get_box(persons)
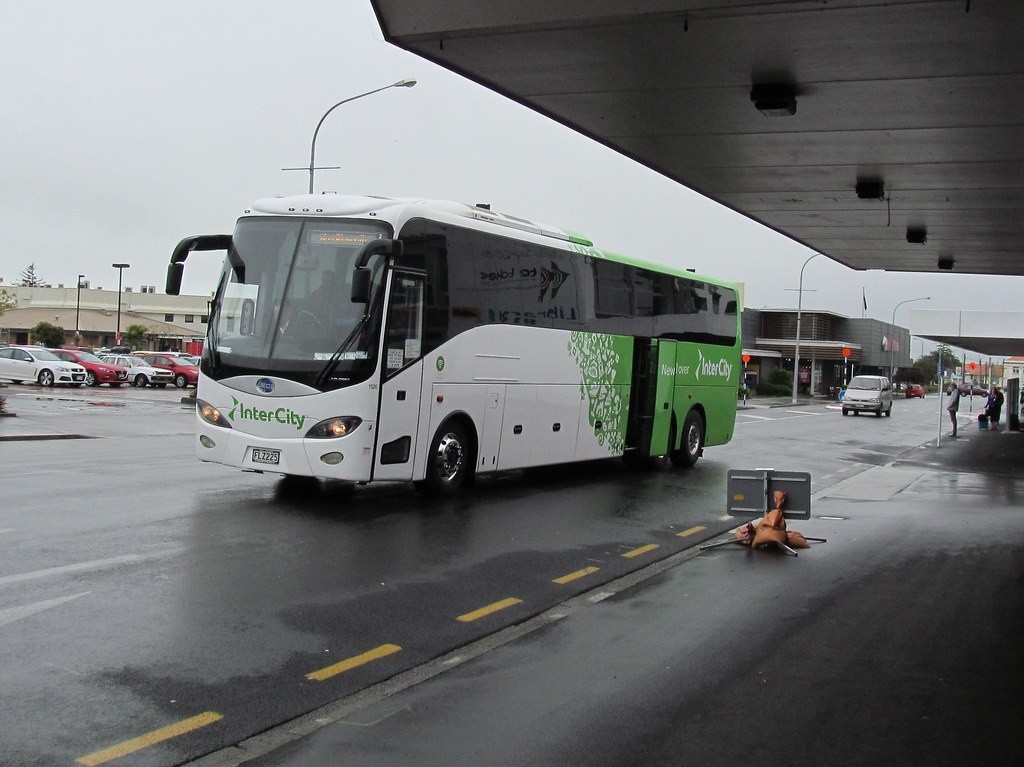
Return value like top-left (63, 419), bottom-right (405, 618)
top-left (947, 382), bottom-right (960, 436)
top-left (984, 387), bottom-right (1001, 431)
top-left (996, 386), bottom-right (1004, 424)
top-left (262, 298), bottom-right (306, 344)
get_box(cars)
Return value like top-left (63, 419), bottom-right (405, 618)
top-left (982, 384), bottom-right (1001, 390)
top-left (97, 356), bottom-right (175, 388)
top-left (959, 384), bottom-right (988, 397)
top-left (59, 347), bottom-right (201, 365)
top-left (47, 348), bottom-right (130, 388)
top-left (0, 346), bottom-right (87, 387)
top-left (140, 353), bottom-right (200, 388)
top-left (947, 386), bottom-right (953, 395)
top-left (906, 384), bottom-right (925, 398)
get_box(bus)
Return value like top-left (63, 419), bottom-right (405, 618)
top-left (165, 193), bottom-right (743, 504)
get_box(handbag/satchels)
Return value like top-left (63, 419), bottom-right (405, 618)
top-left (986, 410), bottom-right (990, 417)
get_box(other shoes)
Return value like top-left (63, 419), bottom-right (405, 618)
top-left (949, 434), bottom-right (956, 436)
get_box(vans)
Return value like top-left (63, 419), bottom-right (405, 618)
top-left (841, 375), bottom-right (893, 415)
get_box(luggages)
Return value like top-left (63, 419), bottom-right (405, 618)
top-left (979, 408), bottom-right (989, 431)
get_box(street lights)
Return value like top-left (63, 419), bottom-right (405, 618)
top-left (76, 275), bottom-right (87, 347)
top-left (890, 297), bottom-right (931, 384)
top-left (309, 77), bottom-right (418, 192)
top-left (113, 263), bottom-right (130, 346)
top-left (913, 337), bottom-right (924, 357)
